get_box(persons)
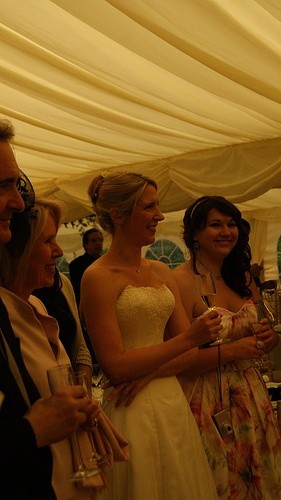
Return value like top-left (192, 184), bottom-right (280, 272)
top-left (0, 118), bottom-right (281, 500)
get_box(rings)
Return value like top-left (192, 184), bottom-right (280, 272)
top-left (255, 341), bottom-right (264, 349)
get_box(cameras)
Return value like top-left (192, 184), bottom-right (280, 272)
top-left (212, 409), bottom-right (234, 437)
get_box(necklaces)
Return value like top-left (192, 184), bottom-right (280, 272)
top-left (109, 248), bottom-right (143, 273)
top-left (196, 257), bottom-right (222, 280)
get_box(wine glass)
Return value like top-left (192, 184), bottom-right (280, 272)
top-left (245, 304), bottom-right (267, 367)
top-left (195, 271), bottom-right (231, 346)
top-left (69, 369), bottom-right (112, 464)
top-left (46, 362), bottom-right (100, 482)
